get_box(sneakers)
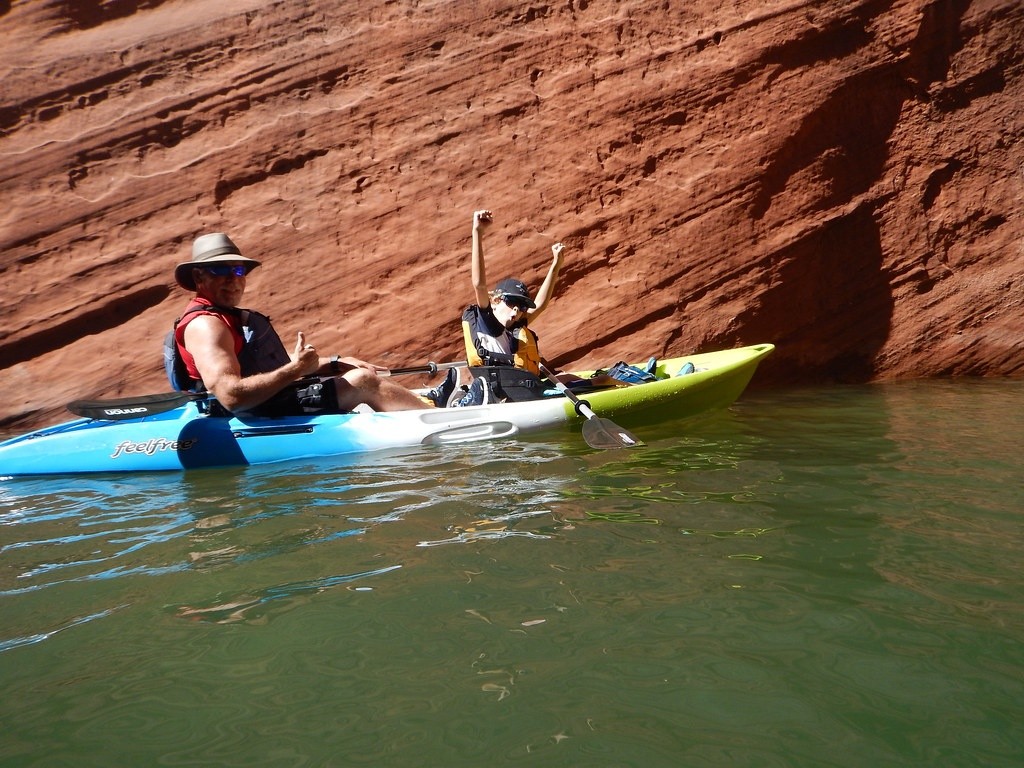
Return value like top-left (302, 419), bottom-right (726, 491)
top-left (421, 366), bottom-right (461, 408)
top-left (451, 376), bottom-right (488, 407)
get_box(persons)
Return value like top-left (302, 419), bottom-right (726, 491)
top-left (164, 232), bottom-right (489, 420)
top-left (460, 209), bottom-right (694, 407)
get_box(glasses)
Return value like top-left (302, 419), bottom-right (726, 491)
top-left (499, 295), bottom-right (527, 313)
top-left (198, 263), bottom-right (248, 276)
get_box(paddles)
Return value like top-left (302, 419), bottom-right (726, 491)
top-left (67, 360), bottom-right (470, 422)
top-left (539, 361), bottom-right (646, 449)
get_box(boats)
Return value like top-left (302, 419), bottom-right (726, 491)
top-left (0, 339), bottom-right (776, 481)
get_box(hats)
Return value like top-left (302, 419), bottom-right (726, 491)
top-left (494, 279), bottom-right (536, 309)
top-left (174, 233), bottom-right (263, 292)
